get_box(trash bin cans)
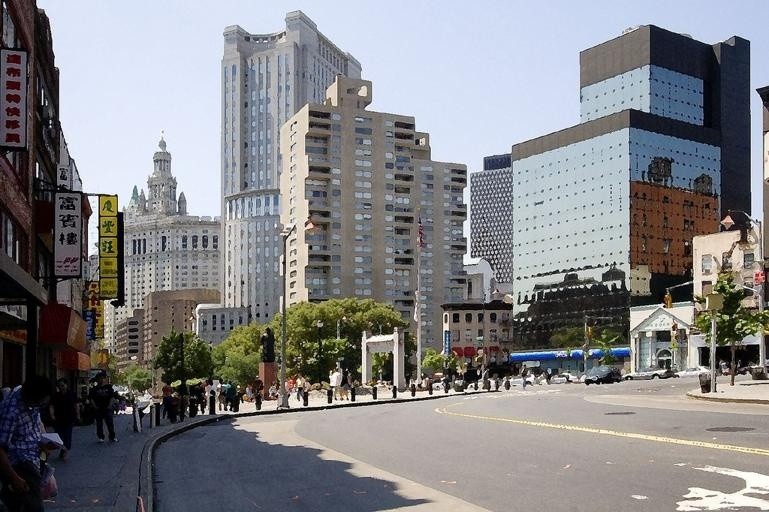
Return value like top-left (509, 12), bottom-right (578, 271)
top-left (698, 372), bottom-right (717, 393)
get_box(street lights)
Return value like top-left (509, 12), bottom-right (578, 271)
top-left (277, 217), bottom-right (315, 408)
top-left (482, 286), bottom-right (501, 371)
top-left (336, 315), bottom-right (391, 381)
top-left (317, 320), bottom-right (324, 383)
top-left (721, 209), bottom-right (767, 377)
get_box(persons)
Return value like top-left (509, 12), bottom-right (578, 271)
top-left (161, 368), bottom-right (350, 418)
top-left (89, 373), bottom-right (119, 442)
top-left (2, 377), bottom-right (81, 510)
top-left (409, 364), bottom-right (530, 391)
top-left (260, 327), bottom-right (275, 362)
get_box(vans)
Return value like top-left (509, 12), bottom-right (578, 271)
top-left (526, 366), bottom-right (551, 382)
top-left (721, 359), bottom-right (747, 376)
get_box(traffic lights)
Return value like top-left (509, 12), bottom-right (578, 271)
top-left (663, 294), bottom-right (672, 308)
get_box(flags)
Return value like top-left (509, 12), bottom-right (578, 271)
top-left (418, 216), bottom-right (426, 248)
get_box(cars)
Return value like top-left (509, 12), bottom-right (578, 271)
top-left (673, 365), bottom-right (718, 377)
top-left (584, 366), bottom-right (622, 384)
top-left (622, 367), bottom-right (675, 380)
top-left (562, 370), bottom-right (573, 375)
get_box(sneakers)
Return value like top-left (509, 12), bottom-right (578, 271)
top-left (97, 438), bottom-right (120, 442)
